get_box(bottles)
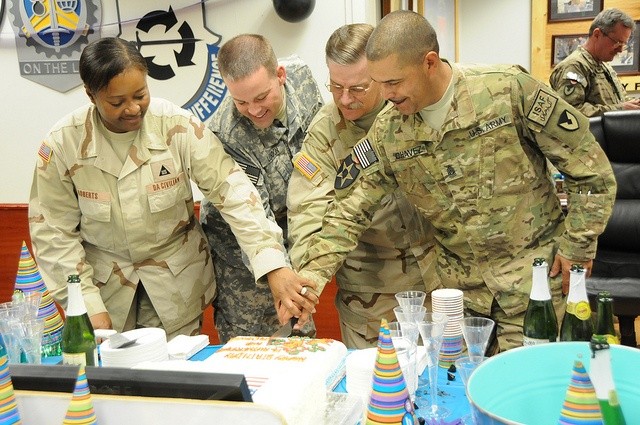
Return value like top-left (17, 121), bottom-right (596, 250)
top-left (522, 258), bottom-right (561, 347)
top-left (61, 274), bottom-right (98, 367)
top-left (591, 292), bottom-right (621, 345)
top-left (559, 264), bottom-right (595, 341)
top-left (588, 338), bottom-right (628, 425)
top-left (598, 291), bottom-right (610, 304)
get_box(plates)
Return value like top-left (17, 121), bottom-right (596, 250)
top-left (100, 326), bottom-right (168, 370)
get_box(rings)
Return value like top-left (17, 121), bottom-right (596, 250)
top-left (299, 286), bottom-right (307, 295)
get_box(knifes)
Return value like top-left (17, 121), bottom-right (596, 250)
top-left (267, 314), bottom-right (301, 346)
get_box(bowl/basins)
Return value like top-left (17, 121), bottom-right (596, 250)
top-left (463, 340), bottom-right (640, 425)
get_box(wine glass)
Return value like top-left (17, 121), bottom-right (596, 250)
top-left (0, 309), bottom-right (27, 366)
top-left (453, 356), bottom-right (487, 425)
top-left (391, 337), bottom-right (429, 412)
top-left (394, 290), bottom-right (426, 313)
top-left (393, 304), bottom-right (427, 344)
top-left (387, 321), bottom-right (430, 388)
top-left (0, 301), bottom-right (25, 312)
top-left (414, 312), bottom-right (451, 419)
top-left (11, 292), bottom-right (43, 321)
top-left (459, 316), bottom-right (495, 363)
top-left (11, 317), bottom-right (45, 365)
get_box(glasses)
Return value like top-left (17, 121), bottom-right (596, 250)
top-left (324, 71), bottom-right (373, 96)
top-left (596, 27), bottom-right (629, 50)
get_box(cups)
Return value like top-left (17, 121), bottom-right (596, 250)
top-left (431, 288), bottom-right (464, 370)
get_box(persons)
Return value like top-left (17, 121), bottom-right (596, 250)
top-left (549, 9), bottom-right (640, 118)
top-left (276, 8), bottom-right (618, 354)
top-left (198, 33), bottom-right (326, 345)
top-left (26, 37), bottom-right (321, 347)
top-left (285, 22), bottom-right (433, 350)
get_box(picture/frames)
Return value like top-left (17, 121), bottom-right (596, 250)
top-left (547, 0), bottom-right (604, 24)
top-left (551, 34), bottom-right (590, 69)
top-left (606, 20), bottom-right (640, 76)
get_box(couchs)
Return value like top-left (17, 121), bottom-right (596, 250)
top-left (588, 112), bottom-right (640, 349)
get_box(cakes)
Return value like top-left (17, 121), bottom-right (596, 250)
top-left (203, 336), bottom-right (348, 396)
